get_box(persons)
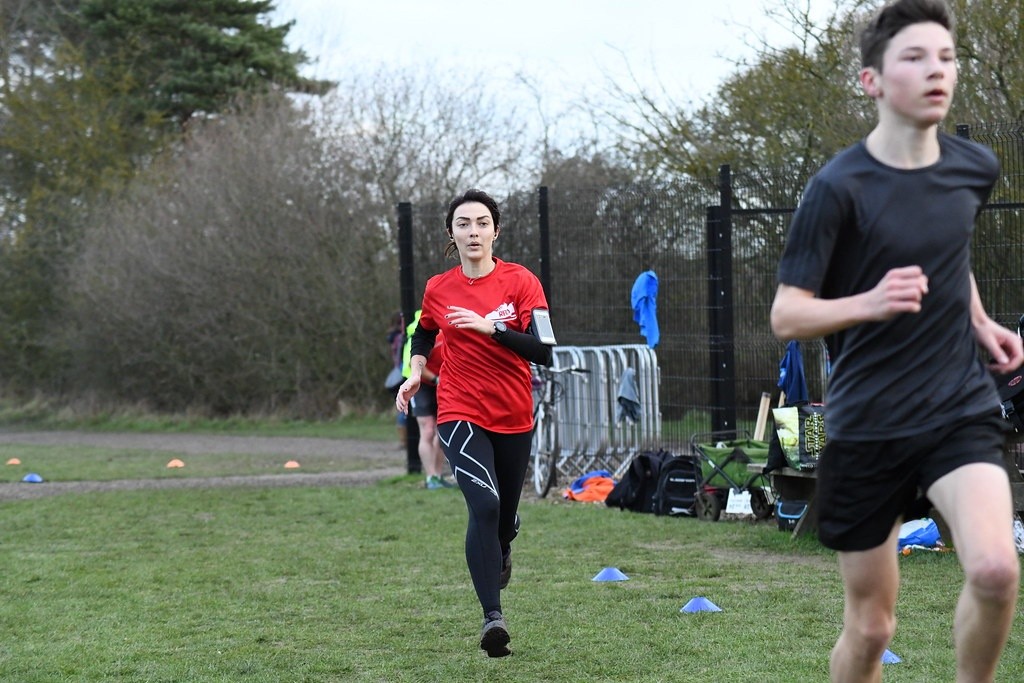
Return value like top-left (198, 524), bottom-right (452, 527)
top-left (388, 189), bottom-right (557, 658)
top-left (770, 1), bottom-right (1023, 682)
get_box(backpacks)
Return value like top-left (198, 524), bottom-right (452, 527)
top-left (605, 446), bottom-right (674, 512)
top-left (652, 456), bottom-right (705, 517)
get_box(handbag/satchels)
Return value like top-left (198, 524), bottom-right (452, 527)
top-left (773, 499), bottom-right (816, 533)
top-left (772, 402), bottom-right (827, 472)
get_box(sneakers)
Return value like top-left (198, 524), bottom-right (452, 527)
top-left (424, 475), bottom-right (443, 489)
top-left (499, 542), bottom-right (513, 590)
top-left (479, 611), bottom-right (512, 657)
top-left (438, 472), bottom-right (462, 490)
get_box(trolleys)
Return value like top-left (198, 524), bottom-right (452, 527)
top-left (690, 428), bottom-right (771, 522)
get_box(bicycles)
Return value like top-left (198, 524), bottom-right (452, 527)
top-left (529, 361), bottom-right (593, 498)
